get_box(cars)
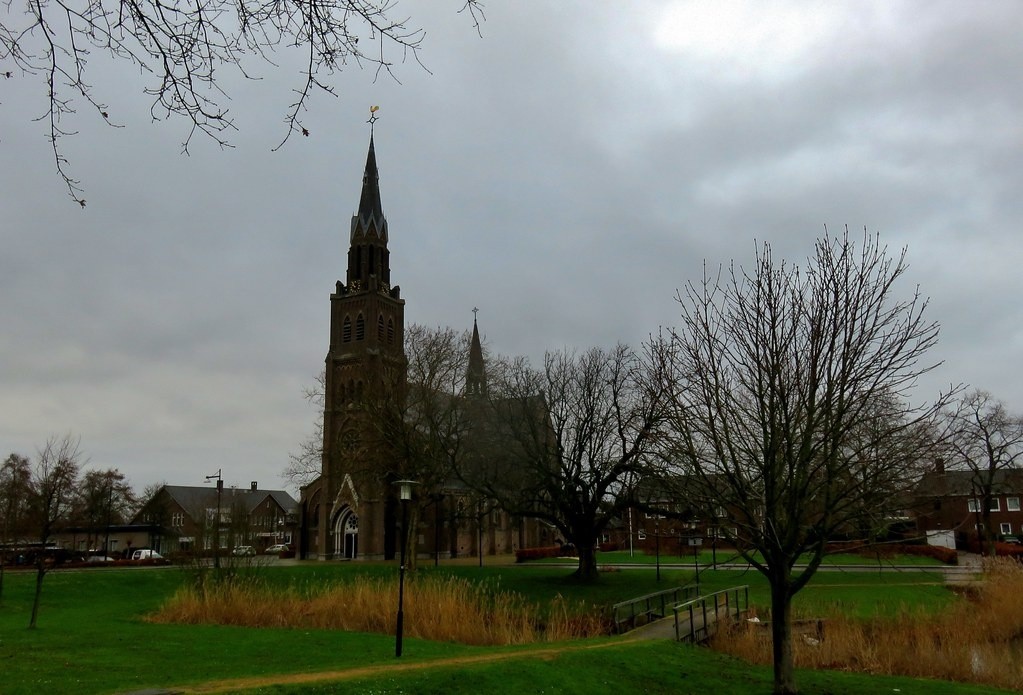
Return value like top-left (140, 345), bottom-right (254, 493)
top-left (1003, 536), bottom-right (1021, 545)
top-left (88, 556), bottom-right (114, 561)
top-left (132, 550), bottom-right (164, 560)
top-left (265, 544), bottom-right (289, 552)
top-left (233, 546), bottom-right (256, 556)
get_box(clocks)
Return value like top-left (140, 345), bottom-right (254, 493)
top-left (351, 280), bottom-right (360, 292)
top-left (380, 281), bottom-right (389, 294)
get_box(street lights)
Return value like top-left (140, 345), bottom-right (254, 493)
top-left (204, 474), bottom-right (223, 566)
top-left (389, 479), bottom-right (420, 657)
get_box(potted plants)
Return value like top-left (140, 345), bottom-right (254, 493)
top-left (279, 550), bottom-right (296, 558)
